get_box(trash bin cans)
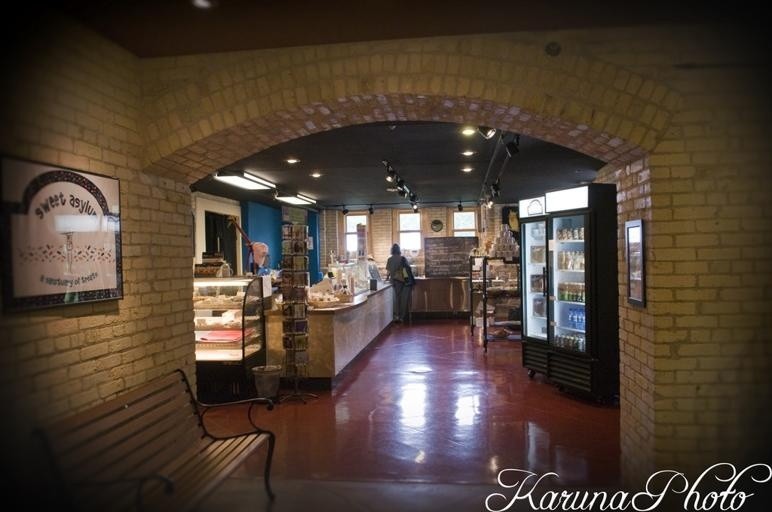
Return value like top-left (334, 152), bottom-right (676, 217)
top-left (251, 364), bottom-right (282, 404)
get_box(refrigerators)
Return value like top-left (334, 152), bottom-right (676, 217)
top-left (544, 182), bottom-right (617, 402)
top-left (517, 194), bottom-right (549, 383)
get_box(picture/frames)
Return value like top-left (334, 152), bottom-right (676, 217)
top-left (624, 218), bottom-right (648, 308)
top-left (1, 150), bottom-right (125, 314)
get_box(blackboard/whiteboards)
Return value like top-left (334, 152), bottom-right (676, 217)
top-left (368, 264), bottom-right (383, 282)
top-left (424, 237), bottom-right (479, 277)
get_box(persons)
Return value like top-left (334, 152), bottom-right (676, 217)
top-left (385, 242), bottom-right (413, 325)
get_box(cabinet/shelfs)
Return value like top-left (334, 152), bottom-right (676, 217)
top-left (467, 255), bottom-right (522, 352)
top-left (392, 277), bottom-right (471, 313)
top-left (193, 278), bottom-right (267, 400)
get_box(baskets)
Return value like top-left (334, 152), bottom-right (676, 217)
top-left (307, 298), bottom-right (340, 308)
top-left (335, 292), bottom-right (354, 303)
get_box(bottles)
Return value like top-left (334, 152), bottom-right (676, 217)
top-left (552, 331), bottom-right (585, 352)
top-left (566, 306), bottom-right (585, 330)
top-left (557, 249), bottom-right (585, 270)
top-left (557, 279), bottom-right (587, 303)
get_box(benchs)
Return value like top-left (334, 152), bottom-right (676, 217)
top-left (36, 369), bottom-right (276, 510)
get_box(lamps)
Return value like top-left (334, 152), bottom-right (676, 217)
top-left (322, 196), bottom-right (480, 215)
top-left (475, 122), bottom-right (518, 210)
top-left (374, 160), bottom-right (422, 216)
top-left (273, 191), bottom-right (317, 206)
top-left (212, 168), bottom-right (280, 191)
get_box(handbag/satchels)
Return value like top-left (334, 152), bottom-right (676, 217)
top-left (402, 263), bottom-right (415, 286)
